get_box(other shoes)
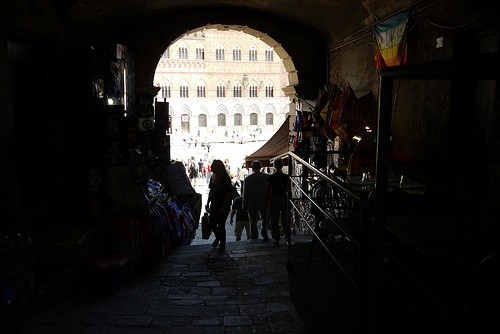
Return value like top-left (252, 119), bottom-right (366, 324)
top-left (220, 239), bottom-right (226, 246)
top-left (285, 236), bottom-right (289, 240)
top-left (262, 234), bottom-right (268, 239)
top-left (212, 239), bottom-right (219, 247)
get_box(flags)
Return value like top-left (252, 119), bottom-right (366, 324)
top-left (293, 12), bottom-right (411, 148)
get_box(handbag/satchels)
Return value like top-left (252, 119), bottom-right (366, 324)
top-left (202, 210), bottom-right (211, 239)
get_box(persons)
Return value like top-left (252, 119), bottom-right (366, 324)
top-left (184, 125), bottom-right (292, 247)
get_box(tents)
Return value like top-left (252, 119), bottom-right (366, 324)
top-left (246, 115), bottom-right (290, 169)
